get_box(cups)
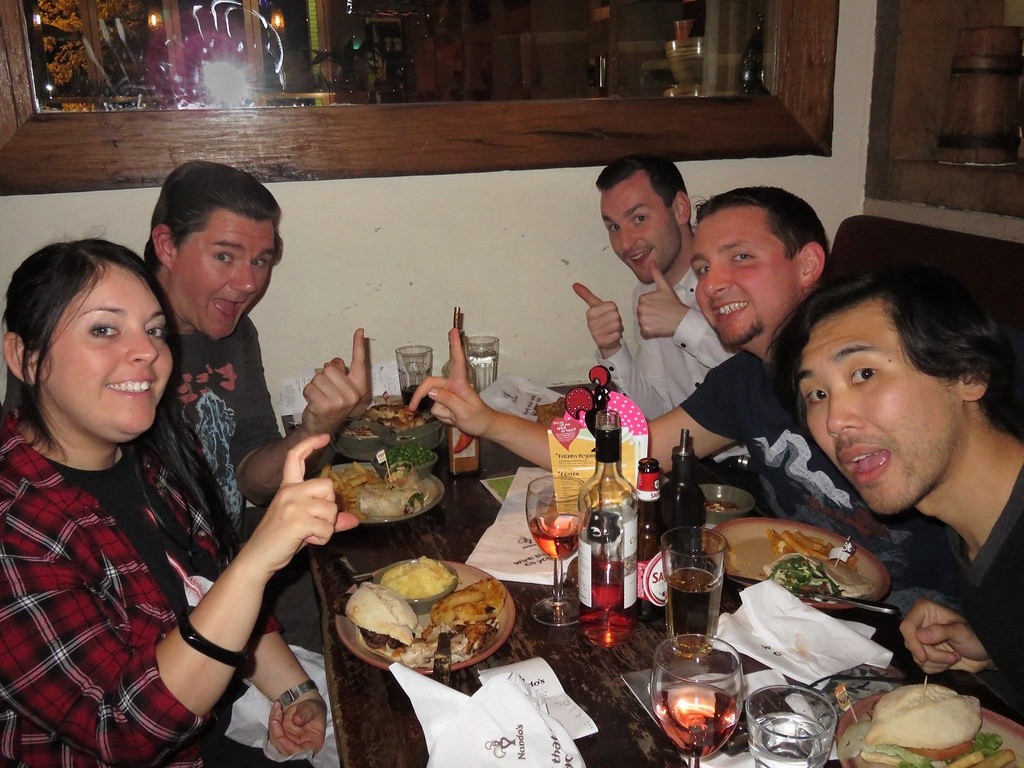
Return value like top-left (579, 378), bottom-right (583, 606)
top-left (466, 335), bottom-right (499, 392)
top-left (396, 345), bottom-right (433, 412)
top-left (661, 528), bottom-right (727, 658)
top-left (745, 684), bottom-right (837, 768)
top-left (596, 54), bottom-right (607, 89)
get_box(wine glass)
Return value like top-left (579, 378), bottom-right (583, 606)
top-left (650, 633), bottom-right (744, 768)
top-left (526, 475), bottom-right (587, 627)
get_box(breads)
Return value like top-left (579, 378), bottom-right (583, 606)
top-left (369, 404), bottom-right (426, 432)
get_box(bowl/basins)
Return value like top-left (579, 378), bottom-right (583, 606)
top-left (372, 558), bottom-right (459, 616)
top-left (664, 37), bottom-right (704, 85)
top-left (336, 421), bottom-right (441, 453)
top-left (372, 447), bottom-right (437, 481)
top-left (696, 483), bottom-right (754, 525)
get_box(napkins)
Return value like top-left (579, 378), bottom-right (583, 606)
top-left (711, 580), bottom-right (894, 690)
top-left (465, 465), bottom-right (580, 586)
top-left (388, 661), bottom-right (588, 768)
top-left (648, 669), bottom-right (840, 768)
top-left (224, 644), bottom-right (340, 768)
top-left (478, 375), bottom-right (566, 423)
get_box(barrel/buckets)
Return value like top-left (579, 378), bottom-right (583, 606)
top-left (938, 26), bottom-right (1024, 162)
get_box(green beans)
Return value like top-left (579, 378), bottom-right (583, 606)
top-left (386, 441), bottom-right (434, 468)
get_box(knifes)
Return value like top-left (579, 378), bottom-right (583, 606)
top-left (432, 633), bottom-right (451, 687)
top-left (728, 575), bottom-right (901, 615)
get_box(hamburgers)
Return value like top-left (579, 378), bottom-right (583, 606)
top-left (836, 683), bottom-right (1002, 768)
top-left (345, 582), bottom-right (424, 661)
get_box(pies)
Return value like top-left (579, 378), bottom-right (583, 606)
top-left (763, 553), bottom-right (876, 597)
top-left (392, 578), bottom-right (507, 669)
top-left (360, 462), bottom-right (430, 515)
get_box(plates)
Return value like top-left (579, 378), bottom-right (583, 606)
top-left (305, 463), bottom-right (445, 524)
top-left (705, 517), bottom-right (892, 614)
top-left (334, 561), bottom-right (516, 675)
top-left (836, 693), bottom-right (1024, 768)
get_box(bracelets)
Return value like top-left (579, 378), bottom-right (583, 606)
top-left (276, 680), bottom-right (318, 707)
top-left (178, 606), bottom-right (246, 667)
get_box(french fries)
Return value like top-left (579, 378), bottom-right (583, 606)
top-left (319, 461), bottom-right (381, 519)
top-left (945, 749), bottom-right (1015, 768)
top-left (768, 528), bottom-right (858, 570)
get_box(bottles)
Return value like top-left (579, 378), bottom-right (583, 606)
top-left (442, 329), bottom-right (481, 475)
top-left (577, 410), bottom-right (639, 649)
top-left (738, 14), bottom-right (770, 96)
top-left (631, 458), bottom-right (668, 623)
top-left (661, 427), bottom-right (706, 569)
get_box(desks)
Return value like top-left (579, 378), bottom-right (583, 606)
top-left (281, 381), bottom-right (1024, 768)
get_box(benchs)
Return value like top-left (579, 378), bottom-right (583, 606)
top-left (827, 216), bottom-right (1024, 405)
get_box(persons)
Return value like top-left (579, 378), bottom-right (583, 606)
top-left (408, 186), bottom-right (971, 620)
top-left (763, 259), bottom-right (1024, 727)
top-left (121, 163), bottom-right (368, 653)
top-left (0, 239), bottom-right (360, 768)
top-left (573, 151), bottom-right (744, 419)
